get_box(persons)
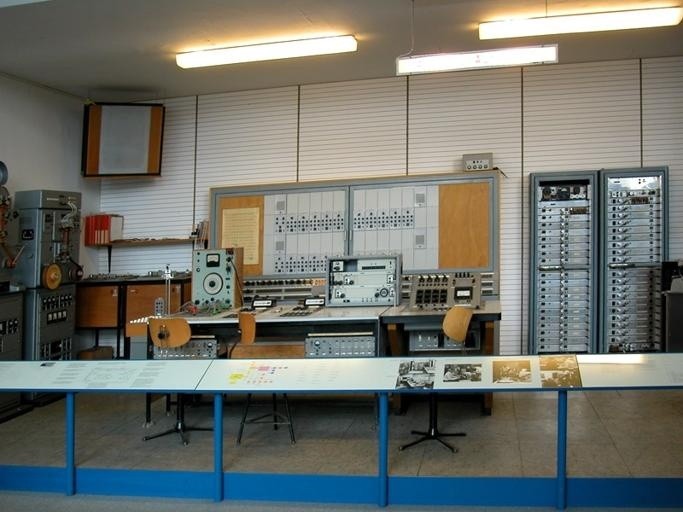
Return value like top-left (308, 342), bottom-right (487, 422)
top-left (443, 366), bottom-right (481, 382)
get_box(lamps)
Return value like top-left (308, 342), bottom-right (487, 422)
top-left (475, 4), bottom-right (682, 43)
top-left (393, 40), bottom-right (561, 81)
top-left (173, 30), bottom-right (362, 73)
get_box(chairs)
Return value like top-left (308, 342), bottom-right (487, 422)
top-left (395, 302), bottom-right (478, 457)
top-left (136, 314), bottom-right (216, 445)
top-left (225, 313), bottom-right (299, 445)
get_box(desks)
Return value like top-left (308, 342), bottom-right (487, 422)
top-left (121, 272), bottom-right (188, 360)
top-left (75, 278), bottom-right (125, 359)
top-left (126, 300), bottom-right (391, 431)
top-left (380, 301), bottom-right (503, 422)
